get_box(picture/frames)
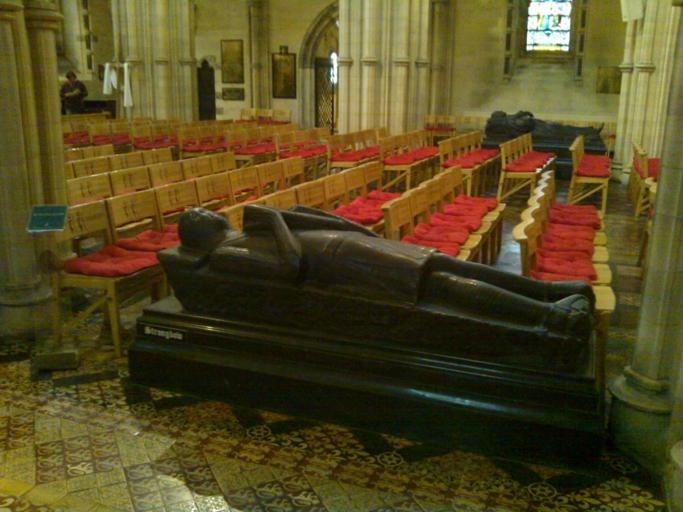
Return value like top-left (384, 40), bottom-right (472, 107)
top-left (221, 88), bottom-right (245, 101)
top-left (221, 39), bottom-right (244, 83)
top-left (272, 46), bottom-right (296, 98)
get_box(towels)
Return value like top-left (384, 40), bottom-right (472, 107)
top-left (122, 62), bottom-right (134, 108)
top-left (102, 62), bottom-right (117, 96)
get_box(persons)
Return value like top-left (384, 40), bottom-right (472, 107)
top-left (59, 70), bottom-right (89, 115)
top-left (179, 203), bottom-right (596, 337)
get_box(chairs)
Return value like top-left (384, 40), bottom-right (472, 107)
top-left (49, 107), bottom-right (662, 359)
